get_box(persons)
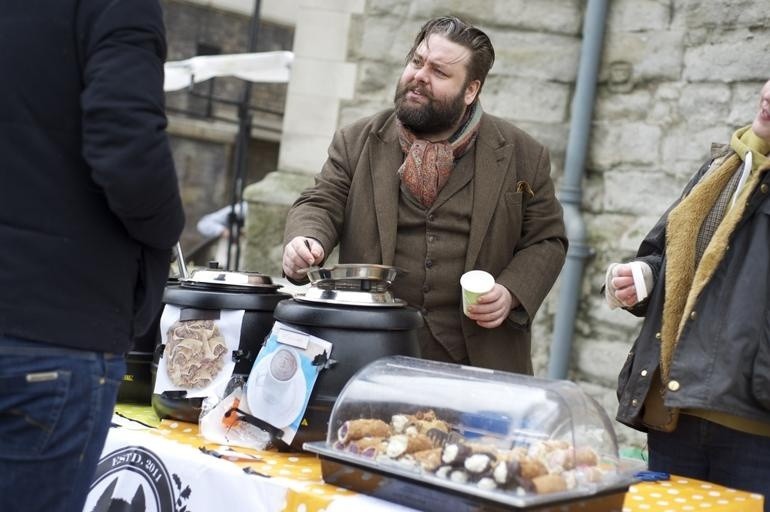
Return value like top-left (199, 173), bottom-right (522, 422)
top-left (603, 77), bottom-right (768, 511)
top-left (0, 0), bottom-right (184, 511)
top-left (281, 14), bottom-right (568, 375)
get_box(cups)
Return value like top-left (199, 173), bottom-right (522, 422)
top-left (255, 345), bottom-right (301, 406)
top-left (460, 270), bottom-right (495, 319)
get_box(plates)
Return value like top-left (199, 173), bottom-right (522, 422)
top-left (246, 352), bottom-right (307, 429)
